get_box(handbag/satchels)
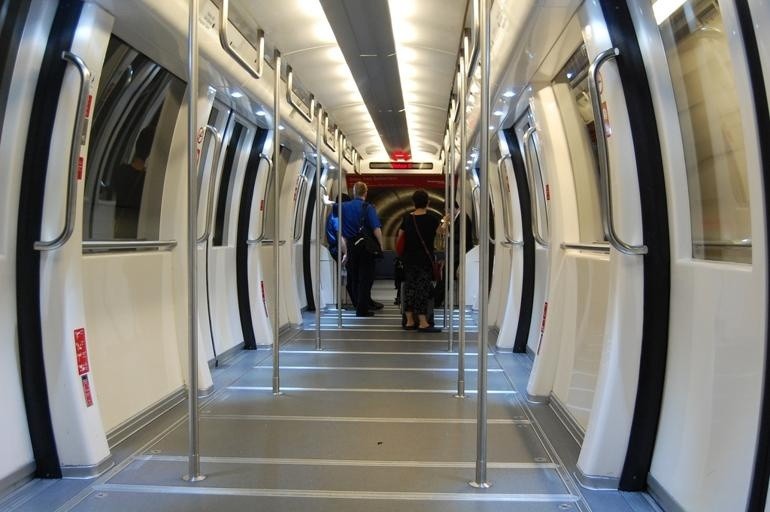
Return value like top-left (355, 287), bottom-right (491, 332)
top-left (432, 260), bottom-right (444, 281)
top-left (358, 203), bottom-right (383, 259)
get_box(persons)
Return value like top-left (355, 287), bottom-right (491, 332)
top-left (434, 198), bottom-right (473, 308)
top-left (398, 190), bottom-right (451, 332)
top-left (322, 181), bottom-right (383, 317)
top-left (326, 192), bottom-right (384, 310)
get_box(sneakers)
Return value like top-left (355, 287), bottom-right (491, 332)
top-left (355, 302), bottom-right (383, 316)
top-left (405, 324), bottom-right (441, 332)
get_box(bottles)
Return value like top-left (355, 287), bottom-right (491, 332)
top-left (341, 265), bottom-right (347, 286)
